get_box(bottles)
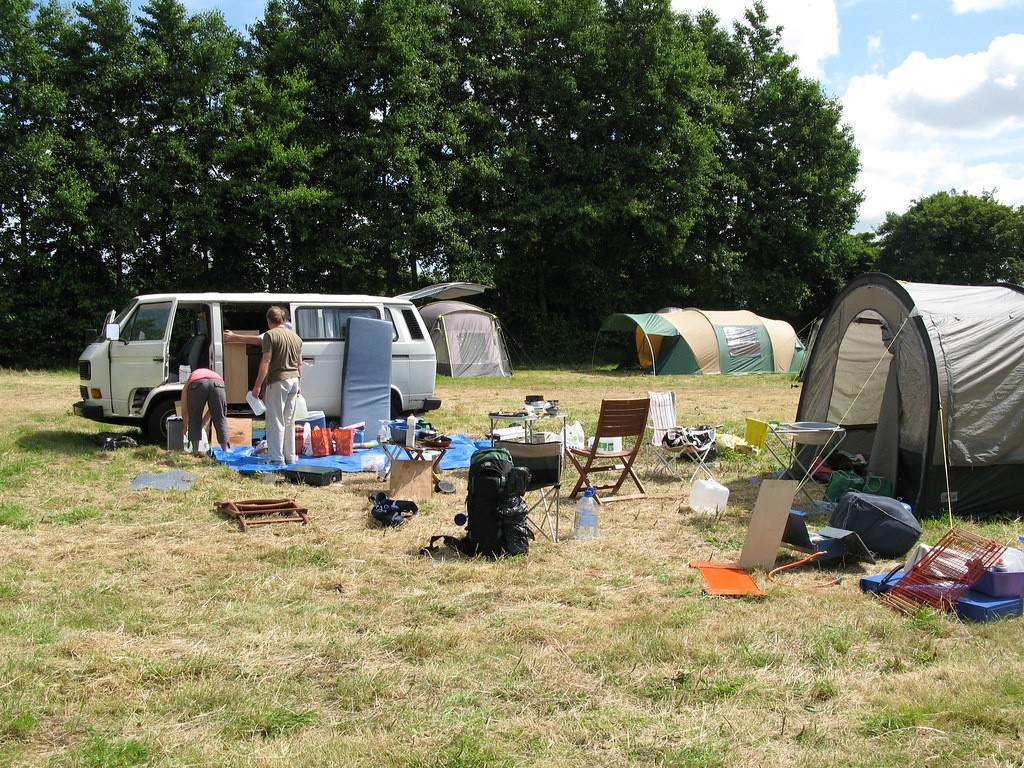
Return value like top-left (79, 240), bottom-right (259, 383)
top-left (575, 486), bottom-right (599, 537)
top-left (245, 391), bottom-right (267, 416)
top-left (183, 428), bottom-right (210, 453)
top-left (982, 558), bottom-right (1008, 572)
top-left (378, 420), bottom-right (388, 442)
top-left (406, 414), bottom-right (415, 447)
top-left (810, 500), bottom-right (838, 515)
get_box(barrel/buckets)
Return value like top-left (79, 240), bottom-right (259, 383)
top-left (598, 437), bottom-right (622, 454)
top-left (179, 365), bottom-right (191, 384)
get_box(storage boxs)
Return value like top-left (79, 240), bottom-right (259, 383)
top-left (223, 330), bottom-right (259, 343)
top-left (285, 466), bottom-right (342, 487)
top-left (532, 433), bottom-right (546, 444)
top-left (965, 559), bottom-right (1024, 598)
top-left (296, 411), bottom-right (326, 430)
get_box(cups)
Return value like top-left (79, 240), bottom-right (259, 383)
top-left (418, 420), bottom-right (432, 441)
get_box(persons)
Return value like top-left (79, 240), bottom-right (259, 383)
top-left (181, 368), bottom-right (231, 456)
top-left (223, 305), bottom-right (303, 466)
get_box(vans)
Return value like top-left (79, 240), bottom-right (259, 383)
top-left (72, 281), bottom-right (493, 447)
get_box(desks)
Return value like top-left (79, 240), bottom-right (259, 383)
top-left (489, 413), bottom-right (569, 472)
top-left (378, 438), bottom-right (456, 493)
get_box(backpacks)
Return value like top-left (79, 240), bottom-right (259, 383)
top-left (463, 446), bottom-right (517, 559)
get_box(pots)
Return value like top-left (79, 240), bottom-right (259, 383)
top-left (389, 422), bottom-right (422, 443)
top-left (433, 471), bottom-right (457, 495)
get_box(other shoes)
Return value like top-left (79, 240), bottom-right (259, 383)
top-left (226, 448), bottom-right (233, 453)
top-left (285, 454), bottom-right (299, 465)
top-left (258, 459), bottom-right (286, 466)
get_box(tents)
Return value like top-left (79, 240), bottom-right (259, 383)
top-left (592, 273), bottom-right (1024, 521)
top-left (416, 300), bottom-right (513, 379)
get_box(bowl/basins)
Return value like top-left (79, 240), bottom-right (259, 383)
top-left (424, 436), bottom-right (451, 447)
top-left (526, 396), bottom-right (544, 415)
top-left (790, 421), bottom-right (840, 445)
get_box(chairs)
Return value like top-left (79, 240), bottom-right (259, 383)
top-left (214, 498), bottom-right (309, 532)
top-left (645, 390), bottom-right (716, 484)
top-left (566, 397), bottom-right (651, 505)
top-left (494, 440), bottom-right (565, 543)
top-left (169, 335), bottom-right (205, 381)
